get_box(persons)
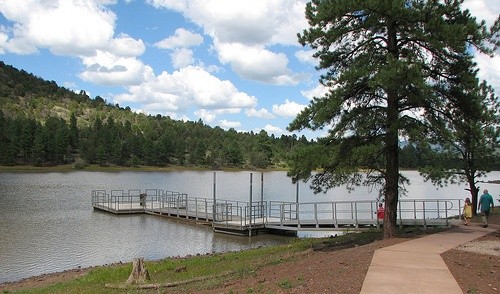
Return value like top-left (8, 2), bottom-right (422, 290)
top-left (375, 203), bottom-right (385, 224)
top-left (462, 197), bottom-right (473, 226)
top-left (478, 189), bottom-right (495, 228)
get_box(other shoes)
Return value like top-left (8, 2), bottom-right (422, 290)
top-left (464, 222), bottom-right (471, 226)
top-left (480, 224), bottom-right (488, 228)
